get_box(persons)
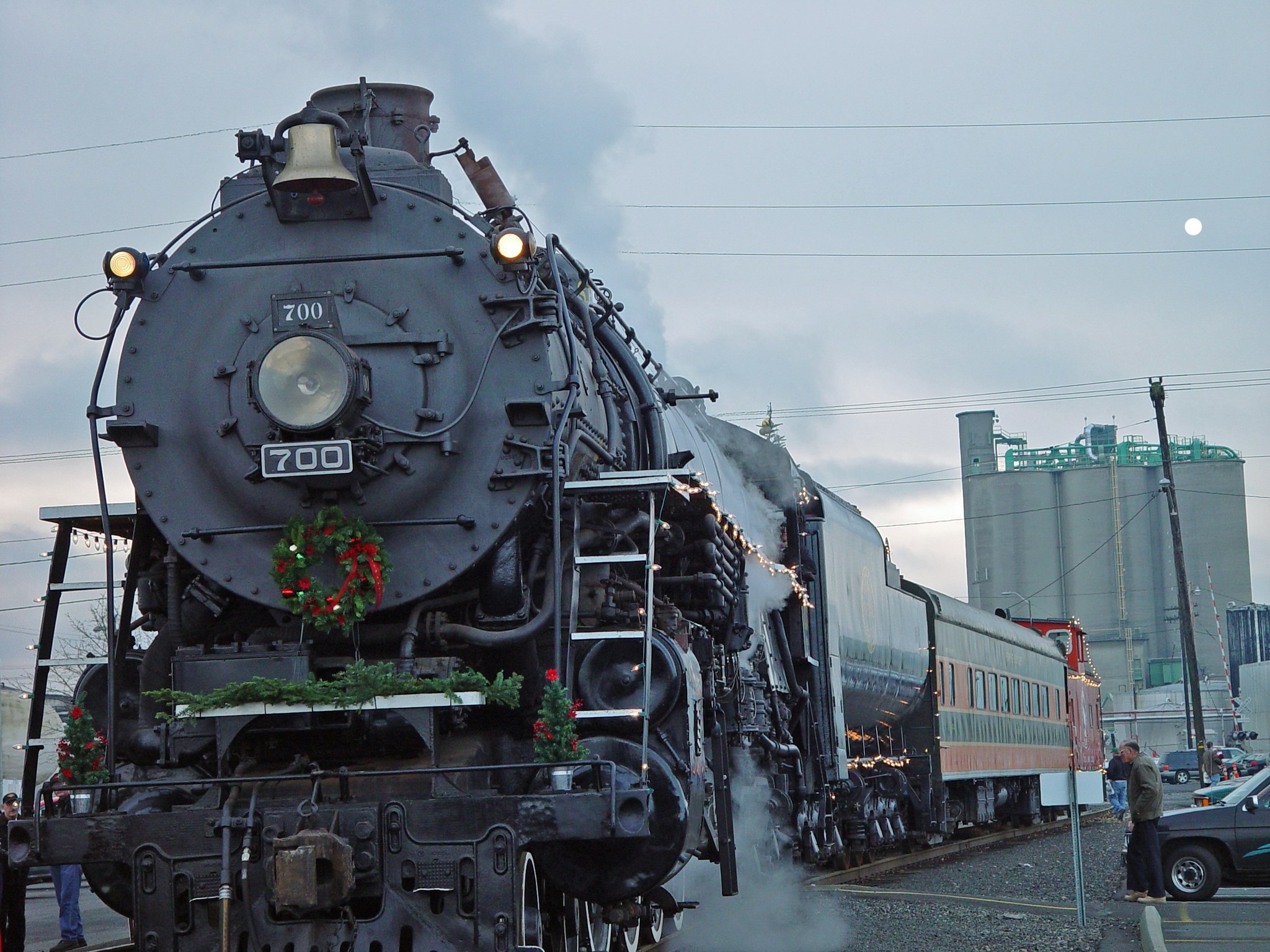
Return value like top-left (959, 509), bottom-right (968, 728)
top-left (1118, 741), bottom-right (1167, 903)
top-left (49, 773), bottom-right (87, 952)
top-left (1107, 746), bottom-right (1128, 821)
top-left (1203, 741), bottom-right (1224, 788)
top-left (0, 792), bottom-right (29, 952)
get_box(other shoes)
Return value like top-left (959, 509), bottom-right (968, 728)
top-left (1124, 891), bottom-right (1149, 902)
top-left (1118, 809), bottom-right (1126, 820)
top-left (1138, 896), bottom-right (1166, 904)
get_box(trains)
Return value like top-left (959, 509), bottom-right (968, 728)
top-left (0, 73), bottom-right (1104, 952)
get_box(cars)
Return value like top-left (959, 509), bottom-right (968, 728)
top-left (1120, 746), bottom-right (1270, 901)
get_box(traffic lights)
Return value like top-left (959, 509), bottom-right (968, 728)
top-left (1237, 730), bottom-right (1258, 740)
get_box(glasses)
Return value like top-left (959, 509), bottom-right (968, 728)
top-left (1117, 749), bottom-right (1127, 754)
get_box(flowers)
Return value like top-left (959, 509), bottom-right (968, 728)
top-left (531, 668), bottom-right (595, 768)
top-left (266, 503), bottom-right (396, 638)
top-left (56, 689), bottom-right (110, 792)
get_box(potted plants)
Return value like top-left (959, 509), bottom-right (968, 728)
top-left (139, 655), bottom-right (526, 725)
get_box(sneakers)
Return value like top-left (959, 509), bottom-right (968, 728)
top-left (78, 938), bottom-right (88, 947)
top-left (49, 939), bottom-right (79, 952)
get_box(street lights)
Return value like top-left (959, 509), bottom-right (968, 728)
top-left (1001, 591), bottom-right (1032, 630)
top-left (1160, 478), bottom-right (1212, 788)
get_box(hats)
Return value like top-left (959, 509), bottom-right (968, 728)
top-left (50, 773), bottom-right (59, 785)
top-left (3, 793), bottom-right (18, 804)
top-left (1110, 747), bottom-right (1119, 754)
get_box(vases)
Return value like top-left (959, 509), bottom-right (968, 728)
top-left (68, 789), bottom-right (93, 814)
top-left (549, 766), bottom-right (577, 794)
